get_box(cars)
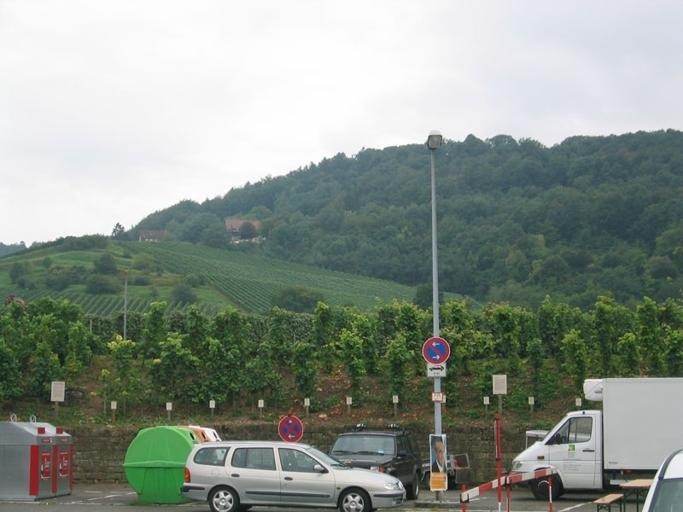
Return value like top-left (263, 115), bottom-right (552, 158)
top-left (640, 450), bottom-right (683, 512)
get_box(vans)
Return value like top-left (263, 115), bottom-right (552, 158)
top-left (180, 441), bottom-right (406, 511)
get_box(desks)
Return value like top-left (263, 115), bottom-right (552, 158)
top-left (618, 477), bottom-right (655, 511)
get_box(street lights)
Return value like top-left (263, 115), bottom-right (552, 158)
top-left (426, 129), bottom-right (448, 496)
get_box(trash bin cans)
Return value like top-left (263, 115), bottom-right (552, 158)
top-left (123, 425), bottom-right (227, 505)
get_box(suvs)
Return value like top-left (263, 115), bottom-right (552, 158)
top-left (326, 424), bottom-right (423, 500)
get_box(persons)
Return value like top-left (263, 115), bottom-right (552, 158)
top-left (432, 440), bottom-right (446, 472)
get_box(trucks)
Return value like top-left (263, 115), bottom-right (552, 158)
top-left (511, 376), bottom-right (683, 500)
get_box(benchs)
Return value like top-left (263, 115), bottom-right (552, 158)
top-left (593, 491), bottom-right (626, 511)
